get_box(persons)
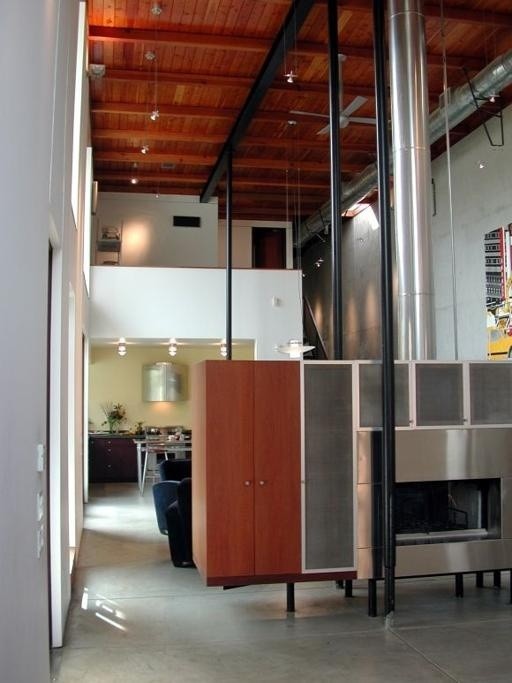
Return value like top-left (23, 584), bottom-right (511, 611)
top-left (486, 308), bottom-right (496, 327)
top-left (503, 303), bottom-right (511, 358)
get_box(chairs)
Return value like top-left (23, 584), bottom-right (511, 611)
top-left (164, 475), bottom-right (196, 568)
top-left (151, 457), bottom-right (191, 535)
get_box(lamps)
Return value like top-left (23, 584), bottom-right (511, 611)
top-left (272, 6), bottom-right (320, 359)
top-left (117, 337), bottom-right (128, 357)
top-left (220, 339), bottom-right (228, 356)
top-left (168, 337), bottom-right (178, 357)
top-left (129, 107), bottom-right (160, 188)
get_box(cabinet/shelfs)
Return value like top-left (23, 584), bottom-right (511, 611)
top-left (188, 356), bottom-right (302, 588)
top-left (87, 429), bottom-right (147, 485)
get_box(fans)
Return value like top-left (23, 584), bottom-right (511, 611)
top-left (288, 49), bottom-right (392, 139)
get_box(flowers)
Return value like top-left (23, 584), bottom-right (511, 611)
top-left (96, 398), bottom-right (130, 434)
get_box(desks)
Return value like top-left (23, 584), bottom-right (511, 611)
top-left (130, 436), bottom-right (192, 495)
